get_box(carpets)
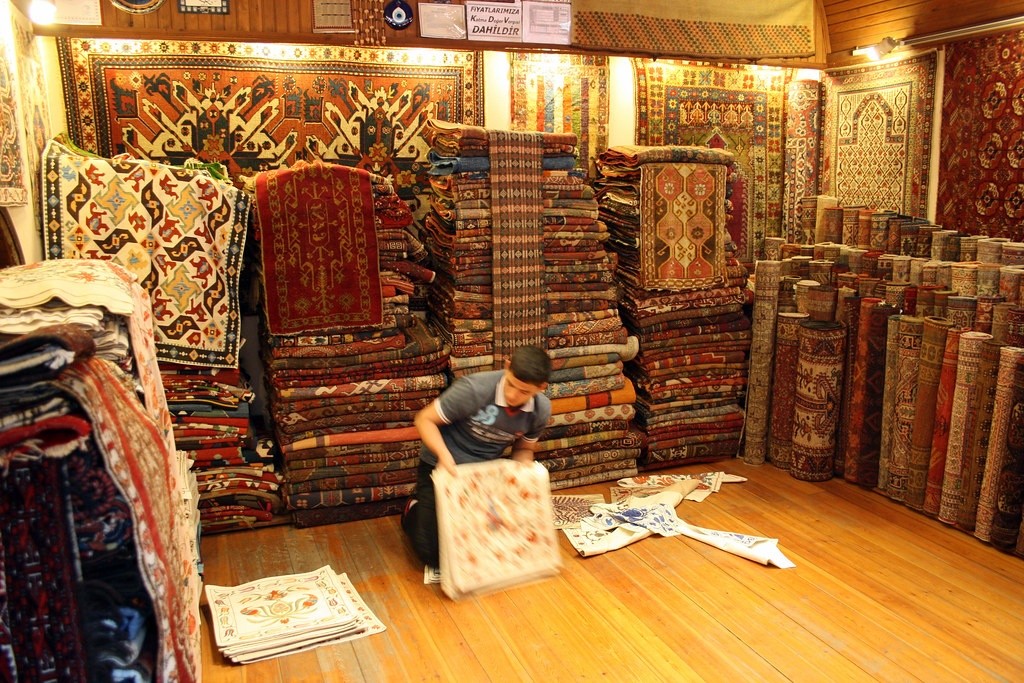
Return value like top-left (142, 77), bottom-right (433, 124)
top-left (570, 0), bottom-right (832, 59)
top-left (257, 174), bottom-right (456, 531)
top-left (818, 48), bottom-right (939, 222)
top-left (734, 194), bottom-right (1024, 560)
top-left (420, 117), bottom-right (650, 493)
top-left (55, 34), bottom-right (485, 192)
top-left (38, 139), bottom-right (253, 370)
top-left (639, 162), bottom-right (730, 292)
top-left (156, 361), bottom-right (286, 537)
top-left (255, 158), bottom-right (384, 337)
top-left (429, 460), bottom-right (563, 605)
top-left (618, 491), bottom-right (684, 509)
top-left (609, 479), bottom-right (700, 504)
top-left (0, 30), bottom-right (32, 209)
top-left (590, 145), bottom-right (759, 471)
top-left (562, 523), bottom-right (655, 557)
top-left (936, 27), bottom-right (1024, 243)
top-left (550, 494), bottom-right (607, 530)
top-left (509, 47), bottom-right (610, 188)
top-left (0, 256), bottom-right (203, 683)
top-left (203, 565), bottom-right (387, 665)
top-left (579, 503), bottom-right (688, 537)
top-left (630, 56), bottom-right (798, 263)
top-left (675, 523), bottom-right (798, 570)
top-left (617, 471), bottom-right (747, 503)
top-left (9, 1), bottom-right (54, 236)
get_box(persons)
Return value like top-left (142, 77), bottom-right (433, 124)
top-left (399, 346), bottom-right (552, 566)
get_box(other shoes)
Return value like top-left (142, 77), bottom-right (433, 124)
top-left (402, 493), bottom-right (417, 529)
